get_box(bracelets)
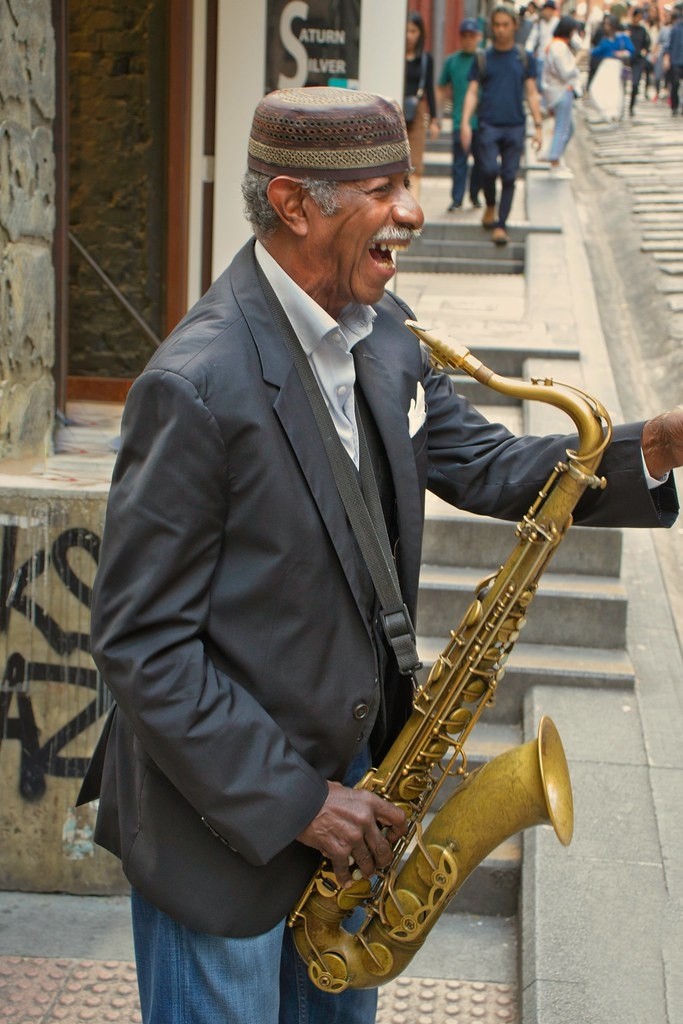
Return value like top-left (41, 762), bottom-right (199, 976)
top-left (535, 124), bottom-right (542, 129)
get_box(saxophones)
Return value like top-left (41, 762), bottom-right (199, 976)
top-left (286, 320), bottom-right (613, 996)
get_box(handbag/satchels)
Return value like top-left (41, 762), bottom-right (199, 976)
top-left (403, 96), bottom-right (419, 127)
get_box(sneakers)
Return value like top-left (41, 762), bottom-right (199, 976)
top-left (549, 165), bottom-right (574, 181)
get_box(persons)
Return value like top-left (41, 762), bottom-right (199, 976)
top-left (92, 88), bottom-right (683, 1024)
top-left (402, 0), bottom-right (683, 249)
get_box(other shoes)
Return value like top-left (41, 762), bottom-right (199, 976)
top-left (448, 203), bottom-right (461, 214)
top-left (491, 228), bottom-right (507, 246)
top-left (468, 202), bottom-right (482, 212)
top-left (483, 205), bottom-right (496, 228)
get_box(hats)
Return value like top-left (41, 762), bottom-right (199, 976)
top-left (460, 18), bottom-right (482, 32)
top-left (248, 87), bottom-right (416, 180)
top-left (543, 0), bottom-right (555, 10)
